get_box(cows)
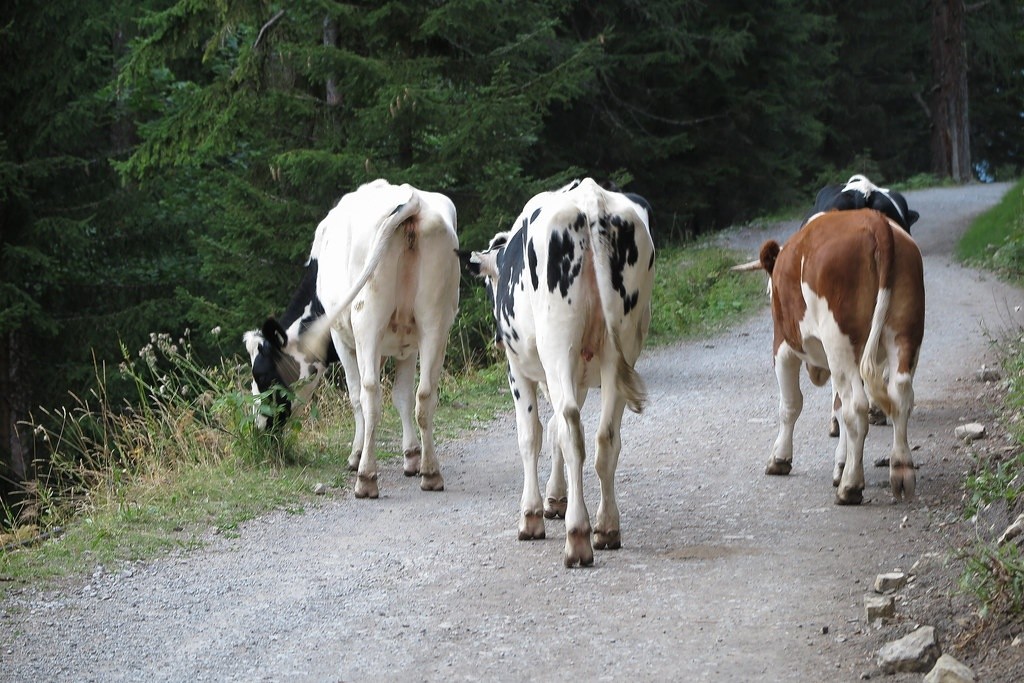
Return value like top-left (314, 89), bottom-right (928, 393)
top-left (760, 172), bottom-right (926, 506)
top-left (242, 179), bottom-right (461, 499)
top-left (454, 177), bottom-right (656, 569)
